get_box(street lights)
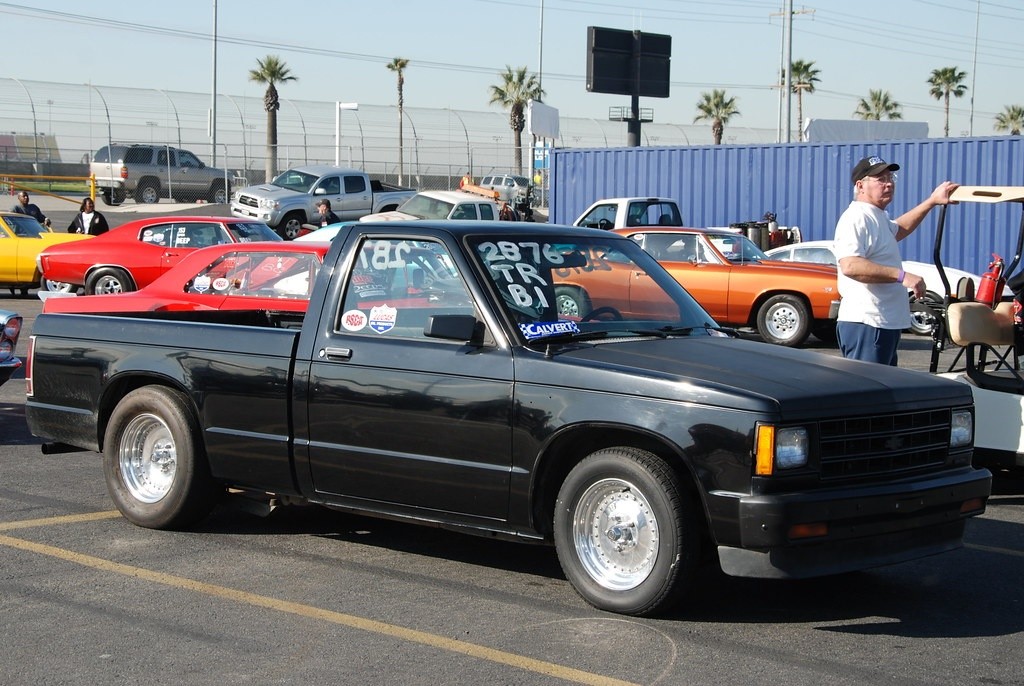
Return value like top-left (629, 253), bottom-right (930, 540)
top-left (335, 100), bottom-right (359, 165)
top-left (10, 75), bottom-right (695, 189)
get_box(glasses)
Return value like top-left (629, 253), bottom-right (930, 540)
top-left (858, 172), bottom-right (898, 185)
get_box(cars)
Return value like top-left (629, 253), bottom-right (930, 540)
top-left (0, 307), bottom-right (23, 389)
top-left (551, 225), bottom-right (843, 346)
top-left (360, 187), bottom-right (527, 224)
top-left (479, 175), bottom-right (529, 202)
top-left (909, 185), bottom-right (1024, 502)
top-left (40, 239), bottom-right (444, 316)
top-left (0, 211), bottom-right (97, 288)
top-left (38, 215), bottom-right (284, 296)
top-left (273, 222), bottom-right (355, 299)
top-left (754, 241), bottom-right (1014, 336)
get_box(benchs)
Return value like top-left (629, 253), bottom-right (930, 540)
top-left (945, 296), bottom-right (1024, 396)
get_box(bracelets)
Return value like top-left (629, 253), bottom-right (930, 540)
top-left (897, 270), bottom-right (905, 284)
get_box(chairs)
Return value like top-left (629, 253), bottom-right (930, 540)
top-left (628, 215), bottom-right (641, 225)
top-left (659, 215), bottom-right (672, 225)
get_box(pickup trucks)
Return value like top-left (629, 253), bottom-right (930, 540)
top-left (229, 165), bottom-right (416, 229)
top-left (23, 219), bottom-right (994, 615)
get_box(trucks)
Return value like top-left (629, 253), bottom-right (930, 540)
top-left (572, 197), bottom-right (802, 251)
top-left (0, 133), bottom-right (63, 164)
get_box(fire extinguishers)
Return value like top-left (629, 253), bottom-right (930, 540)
top-left (976, 253), bottom-right (1005, 312)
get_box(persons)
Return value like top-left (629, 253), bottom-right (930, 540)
top-left (316, 199), bottom-right (341, 229)
top-left (834, 156), bottom-right (961, 367)
top-left (9, 191), bottom-right (52, 295)
top-left (68, 197), bottom-right (109, 237)
top-left (533, 170), bottom-right (543, 185)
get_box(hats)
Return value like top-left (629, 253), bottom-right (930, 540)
top-left (316, 199), bottom-right (331, 209)
top-left (851, 156), bottom-right (900, 185)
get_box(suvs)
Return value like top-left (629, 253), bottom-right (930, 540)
top-left (85, 143), bottom-right (231, 206)
